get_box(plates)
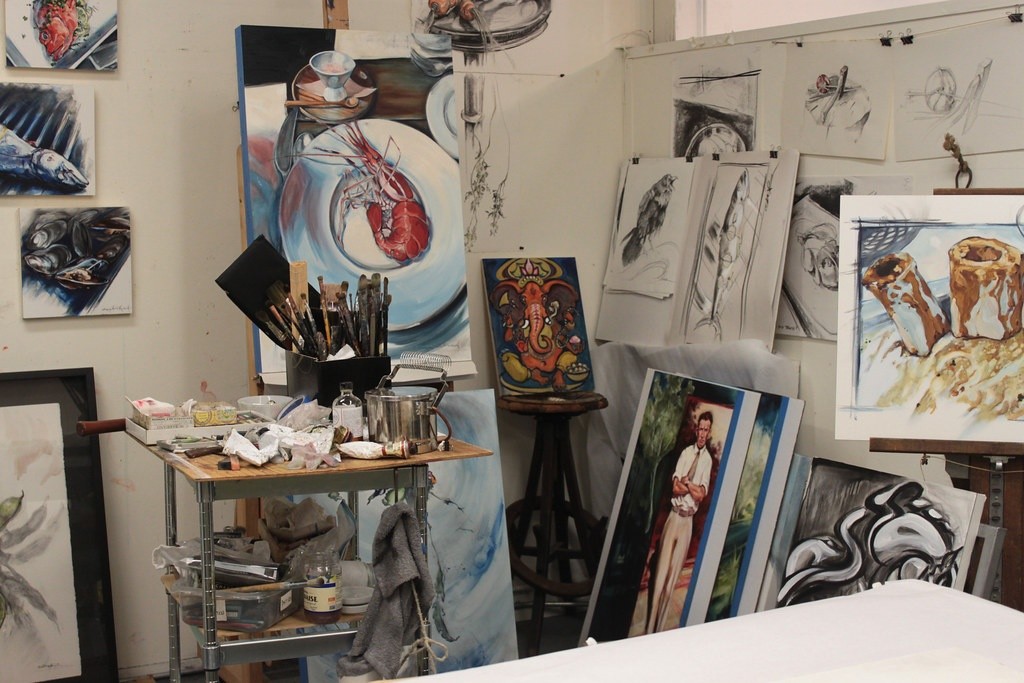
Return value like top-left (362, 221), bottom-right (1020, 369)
top-left (341, 606), bottom-right (367, 614)
top-left (342, 586), bottom-right (375, 604)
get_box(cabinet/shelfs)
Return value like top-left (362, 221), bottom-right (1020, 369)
top-left (125, 428), bottom-right (494, 682)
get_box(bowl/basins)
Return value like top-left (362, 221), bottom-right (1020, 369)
top-left (237, 395), bottom-right (293, 419)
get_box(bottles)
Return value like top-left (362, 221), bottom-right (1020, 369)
top-left (332, 382), bottom-right (363, 442)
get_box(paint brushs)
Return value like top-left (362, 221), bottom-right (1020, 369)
top-left (217, 578), bottom-right (324, 601)
top-left (267, 273), bottom-right (392, 358)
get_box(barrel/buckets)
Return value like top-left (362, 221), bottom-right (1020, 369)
top-left (364, 364), bottom-right (448, 453)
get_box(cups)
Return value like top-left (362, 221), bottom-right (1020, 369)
top-left (302, 549), bottom-right (340, 623)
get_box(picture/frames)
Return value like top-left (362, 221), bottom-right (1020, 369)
top-left (0, 367), bottom-right (119, 683)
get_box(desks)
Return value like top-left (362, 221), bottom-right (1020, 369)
top-left (386, 581), bottom-right (1024, 683)
top-left (496, 395), bottom-right (609, 659)
